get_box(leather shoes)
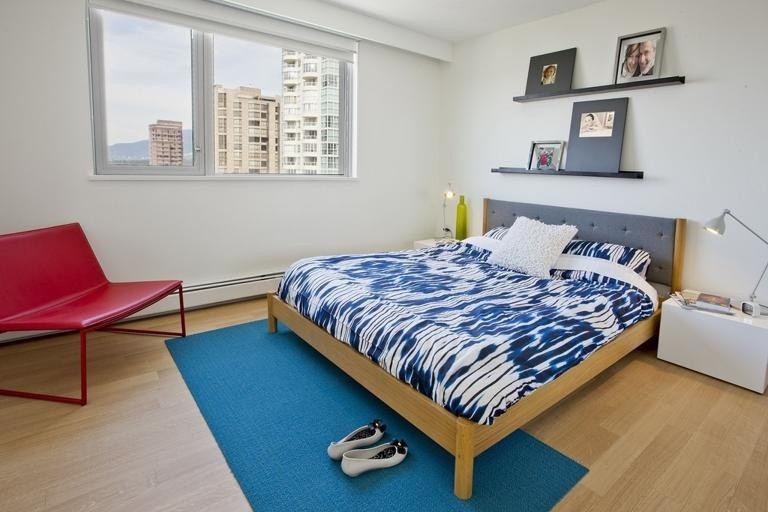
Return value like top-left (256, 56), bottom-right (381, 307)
top-left (340, 438), bottom-right (408, 478)
top-left (327, 419), bottom-right (387, 460)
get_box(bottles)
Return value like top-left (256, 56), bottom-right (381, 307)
top-left (455, 195), bottom-right (467, 240)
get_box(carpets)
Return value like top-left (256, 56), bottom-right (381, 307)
top-left (163, 318), bottom-right (592, 512)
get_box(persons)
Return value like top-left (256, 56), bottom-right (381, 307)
top-left (541, 64), bottom-right (556, 86)
top-left (620, 42), bottom-right (639, 77)
top-left (638, 41), bottom-right (657, 76)
top-left (538, 147), bottom-right (548, 169)
top-left (581, 113), bottom-right (604, 133)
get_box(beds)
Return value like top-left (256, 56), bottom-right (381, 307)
top-left (265, 197), bottom-right (687, 503)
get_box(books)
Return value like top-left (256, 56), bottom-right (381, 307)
top-left (696, 291), bottom-right (731, 314)
top-left (680, 289), bottom-right (701, 307)
top-left (669, 293), bottom-right (690, 309)
top-left (675, 291), bottom-right (695, 308)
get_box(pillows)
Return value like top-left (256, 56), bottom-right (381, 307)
top-left (460, 214), bottom-right (654, 292)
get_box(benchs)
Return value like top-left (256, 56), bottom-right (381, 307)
top-left (1, 223), bottom-right (187, 405)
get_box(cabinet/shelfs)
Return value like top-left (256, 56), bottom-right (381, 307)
top-left (488, 74), bottom-right (686, 179)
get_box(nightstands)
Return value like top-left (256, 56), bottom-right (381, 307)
top-left (657, 287), bottom-right (768, 395)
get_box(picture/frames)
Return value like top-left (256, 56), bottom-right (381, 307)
top-left (610, 26), bottom-right (668, 84)
top-left (526, 140), bottom-right (567, 171)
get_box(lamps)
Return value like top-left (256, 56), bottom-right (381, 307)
top-left (435, 182), bottom-right (454, 240)
top-left (703, 208), bottom-right (768, 315)
